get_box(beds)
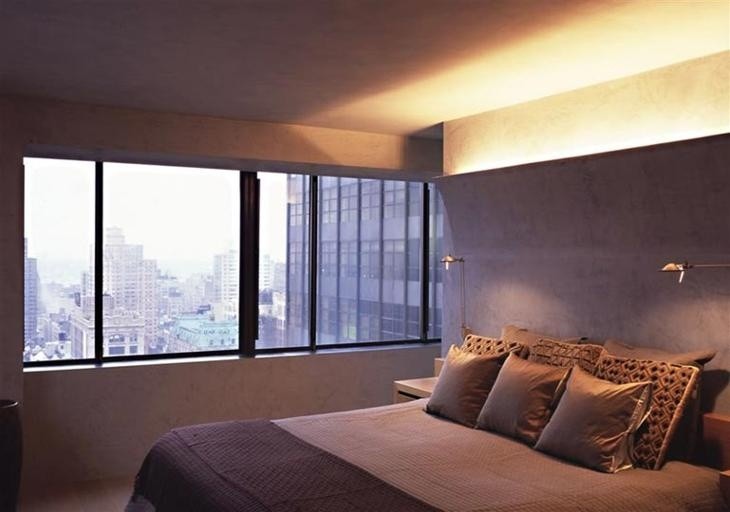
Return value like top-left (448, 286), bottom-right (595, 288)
top-left (145, 399), bottom-right (730, 512)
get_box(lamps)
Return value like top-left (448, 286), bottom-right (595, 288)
top-left (656, 260), bottom-right (730, 275)
top-left (439, 253), bottom-right (472, 339)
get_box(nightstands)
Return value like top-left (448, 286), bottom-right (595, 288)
top-left (392, 358), bottom-right (445, 404)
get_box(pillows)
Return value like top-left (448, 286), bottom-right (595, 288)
top-left (524, 337), bottom-right (606, 375)
top-left (422, 343), bottom-right (509, 430)
top-left (461, 334), bottom-right (530, 359)
top-left (595, 354), bottom-right (704, 474)
top-left (474, 351), bottom-right (574, 449)
top-left (531, 363), bottom-right (655, 474)
top-left (603, 337), bottom-right (718, 465)
top-left (499, 327), bottom-right (582, 353)
